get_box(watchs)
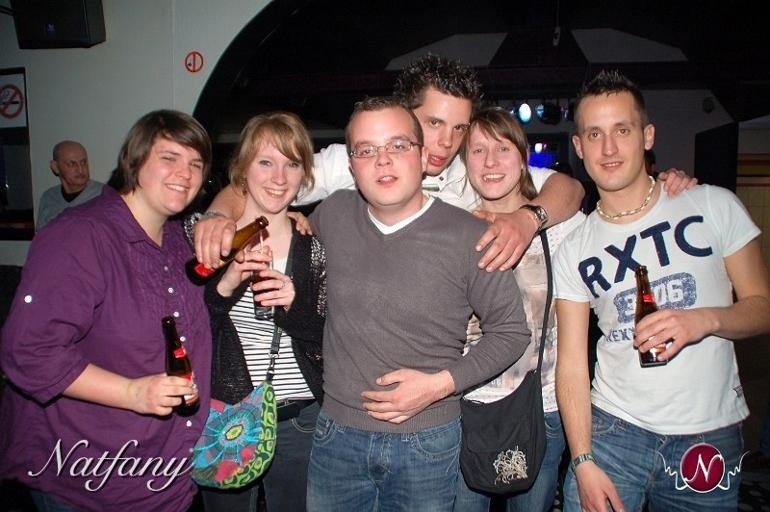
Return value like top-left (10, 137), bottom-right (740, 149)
top-left (518, 203), bottom-right (548, 230)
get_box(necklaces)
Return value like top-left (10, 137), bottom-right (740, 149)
top-left (595, 172), bottom-right (656, 220)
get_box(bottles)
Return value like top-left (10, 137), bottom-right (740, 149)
top-left (183, 215), bottom-right (269, 288)
top-left (633, 265), bottom-right (668, 368)
top-left (162, 316), bottom-right (200, 417)
top-left (251, 230), bottom-right (274, 319)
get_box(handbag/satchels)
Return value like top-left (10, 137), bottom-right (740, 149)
top-left (188, 383), bottom-right (277, 489)
top-left (462, 370), bottom-right (546, 495)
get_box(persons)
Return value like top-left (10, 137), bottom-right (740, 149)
top-left (452, 108), bottom-right (571, 512)
top-left (0, 111), bottom-right (315, 511)
top-left (192, 51), bottom-right (587, 272)
top-left (35, 141), bottom-right (106, 237)
top-left (552, 65), bottom-right (769, 512)
top-left (306, 98), bottom-right (532, 512)
top-left (195, 115), bottom-right (327, 512)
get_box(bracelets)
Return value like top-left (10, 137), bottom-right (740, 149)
top-left (569, 453), bottom-right (597, 472)
top-left (194, 211), bottom-right (224, 220)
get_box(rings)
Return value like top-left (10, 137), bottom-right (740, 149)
top-left (282, 281), bottom-right (284, 284)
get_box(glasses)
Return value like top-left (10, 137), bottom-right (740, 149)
top-left (351, 138), bottom-right (420, 156)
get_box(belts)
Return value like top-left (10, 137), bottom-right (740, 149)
top-left (276, 400), bottom-right (319, 423)
